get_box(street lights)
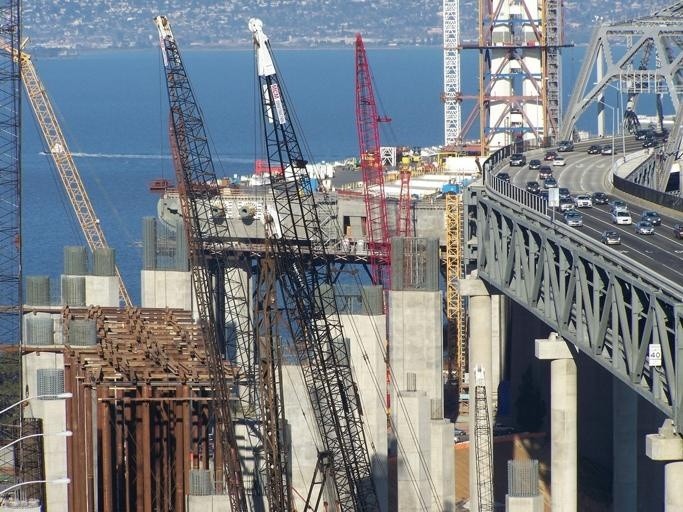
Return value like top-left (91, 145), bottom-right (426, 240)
top-left (582, 98), bottom-right (616, 185)
top-left (410, 193), bottom-right (418, 238)
top-left (592, 82), bottom-right (626, 164)
top-left (0, 478), bottom-right (70, 494)
top-left (0, 428), bottom-right (72, 450)
top-left (535, 76), bottom-right (550, 149)
top-left (0, 390), bottom-right (72, 415)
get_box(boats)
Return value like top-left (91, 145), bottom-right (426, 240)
top-left (148, 178), bottom-right (171, 192)
top-left (41, 146), bottom-right (65, 155)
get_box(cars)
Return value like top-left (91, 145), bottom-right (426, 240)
top-left (497, 129), bottom-right (682, 245)
top-left (454, 428), bottom-right (468, 443)
top-left (492, 421), bottom-right (513, 435)
